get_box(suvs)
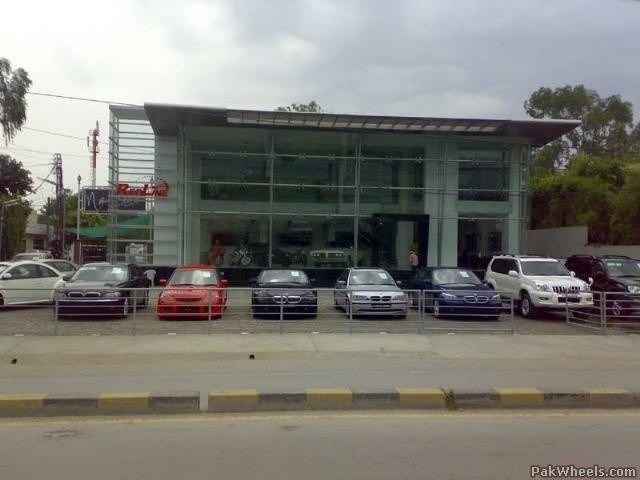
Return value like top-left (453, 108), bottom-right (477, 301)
top-left (482, 252), bottom-right (596, 324)
top-left (561, 253), bottom-right (640, 321)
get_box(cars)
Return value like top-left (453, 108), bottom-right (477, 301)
top-left (404, 266), bottom-right (503, 320)
top-left (0, 260), bottom-right (69, 312)
top-left (330, 266), bottom-right (409, 320)
top-left (53, 261), bottom-right (151, 319)
top-left (156, 263), bottom-right (230, 320)
top-left (287, 217), bottom-right (314, 243)
top-left (13, 246), bottom-right (83, 281)
top-left (246, 269), bottom-right (321, 318)
top-left (310, 241), bottom-right (390, 267)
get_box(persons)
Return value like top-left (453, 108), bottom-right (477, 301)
top-left (410, 249), bottom-right (419, 269)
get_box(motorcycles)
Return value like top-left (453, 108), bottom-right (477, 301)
top-left (229, 247), bottom-right (253, 265)
top-left (205, 245), bottom-right (229, 267)
